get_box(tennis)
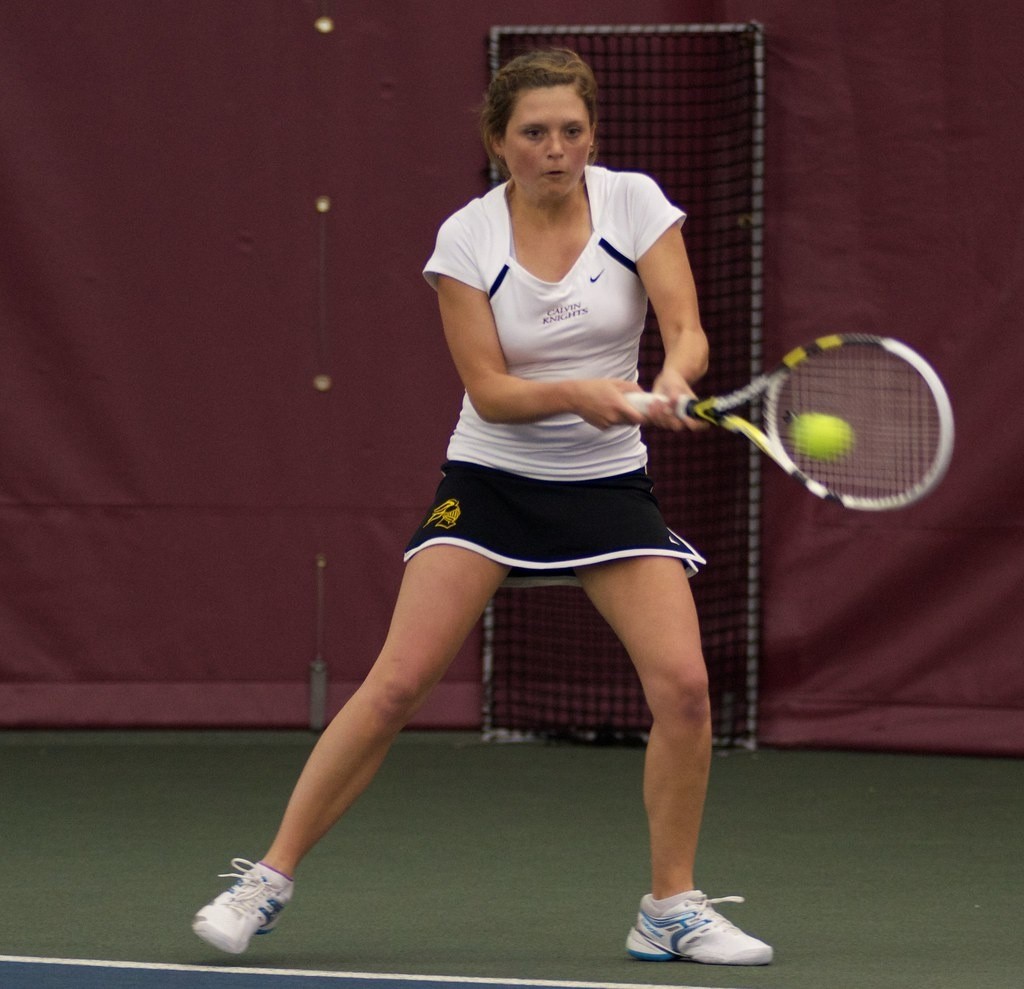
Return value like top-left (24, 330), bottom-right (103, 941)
top-left (789, 409), bottom-right (855, 461)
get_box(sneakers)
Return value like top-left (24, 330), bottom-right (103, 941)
top-left (192, 858), bottom-right (295, 953)
top-left (625, 890), bottom-right (773, 965)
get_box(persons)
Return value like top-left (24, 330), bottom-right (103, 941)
top-left (188, 48), bottom-right (778, 964)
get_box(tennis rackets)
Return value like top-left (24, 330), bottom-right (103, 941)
top-left (624, 333), bottom-right (957, 513)
